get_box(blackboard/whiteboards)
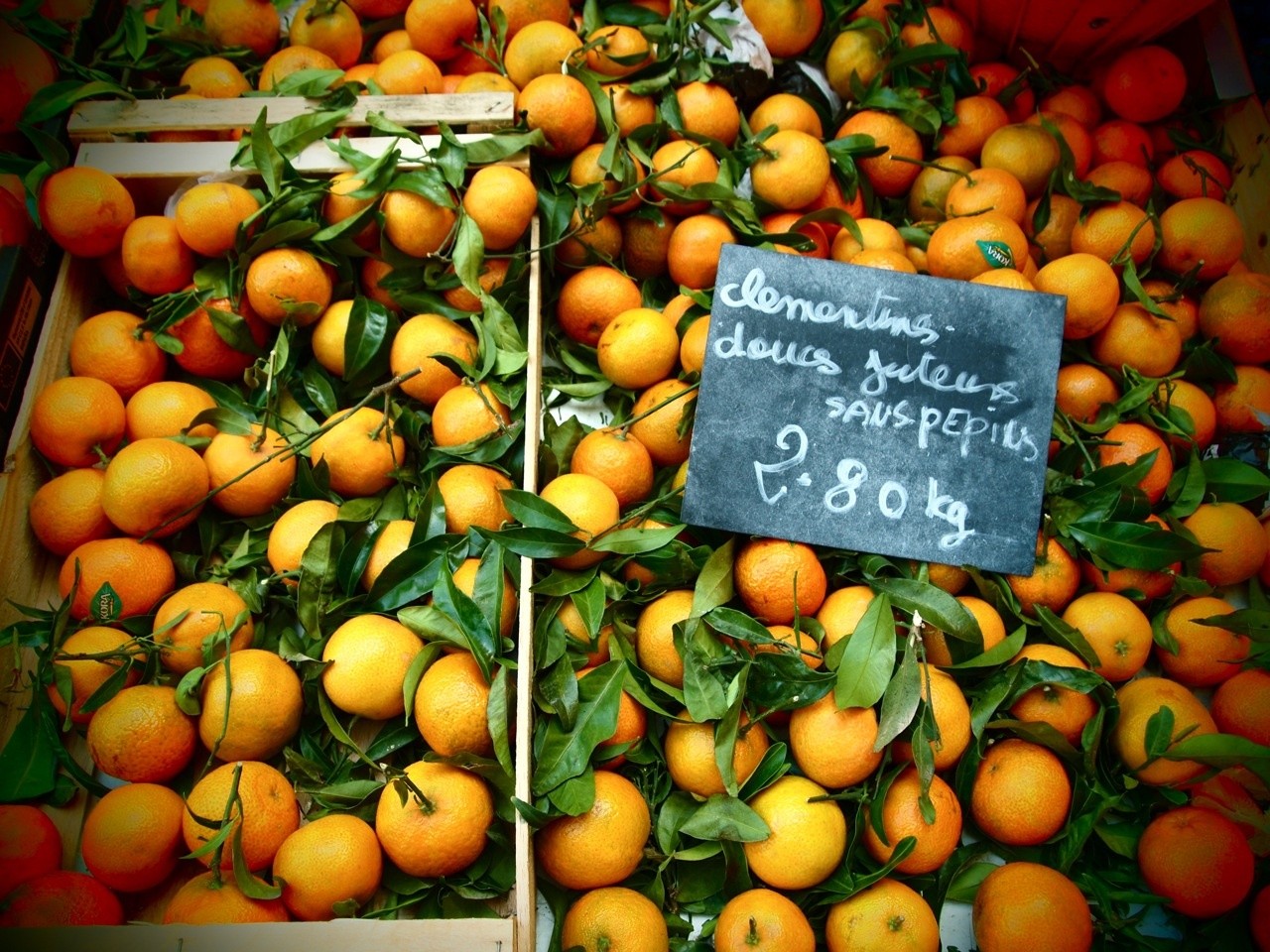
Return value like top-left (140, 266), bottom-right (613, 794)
top-left (678, 244), bottom-right (1066, 574)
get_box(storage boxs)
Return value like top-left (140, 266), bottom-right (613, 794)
top-left (0, 90), bottom-right (539, 952)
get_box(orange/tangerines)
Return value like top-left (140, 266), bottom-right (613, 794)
top-left (0, 0), bottom-right (1269, 951)
